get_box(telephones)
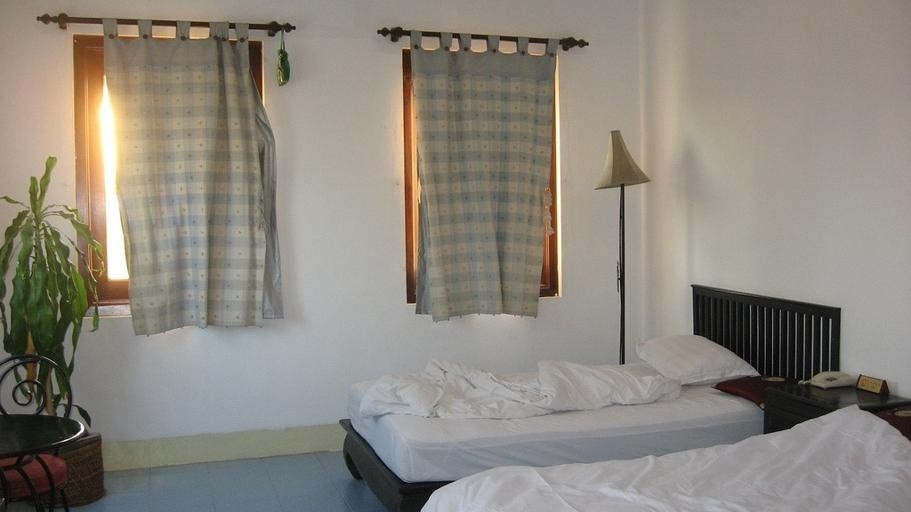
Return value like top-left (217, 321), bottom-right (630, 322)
top-left (808, 371), bottom-right (855, 391)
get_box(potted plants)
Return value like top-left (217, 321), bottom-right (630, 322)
top-left (0, 153), bottom-right (107, 506)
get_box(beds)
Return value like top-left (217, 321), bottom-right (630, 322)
top-left (335, 282), bottom-right (843, 512)
top-left (416, 403), bottom-right (911, 512)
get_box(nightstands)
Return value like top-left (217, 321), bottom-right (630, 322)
top-left (763, 380), bottom-right (910, 435)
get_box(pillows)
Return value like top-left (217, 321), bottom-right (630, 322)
top-left (714, 374), bottom-right (799, 411)
top-left (634, 331), bottom-right (761, 387)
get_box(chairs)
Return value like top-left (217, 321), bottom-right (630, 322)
top-left (2, 352), bottom-right (75, 512)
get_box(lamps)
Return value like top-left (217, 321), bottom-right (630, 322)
top-left (593, 129), bottom-right (651, 364)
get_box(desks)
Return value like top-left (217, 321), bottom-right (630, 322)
top-left (0, 412), bottom-right (85, 512)
